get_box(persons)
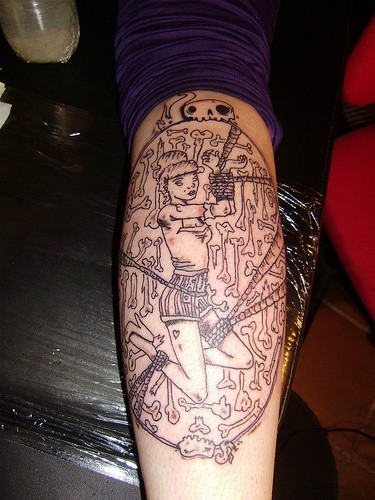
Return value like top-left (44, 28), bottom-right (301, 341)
top-left (111, 0), bottom-right (290, 500)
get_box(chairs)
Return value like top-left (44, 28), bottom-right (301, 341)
top-left (319, 14), bottom-right (375, 330)
